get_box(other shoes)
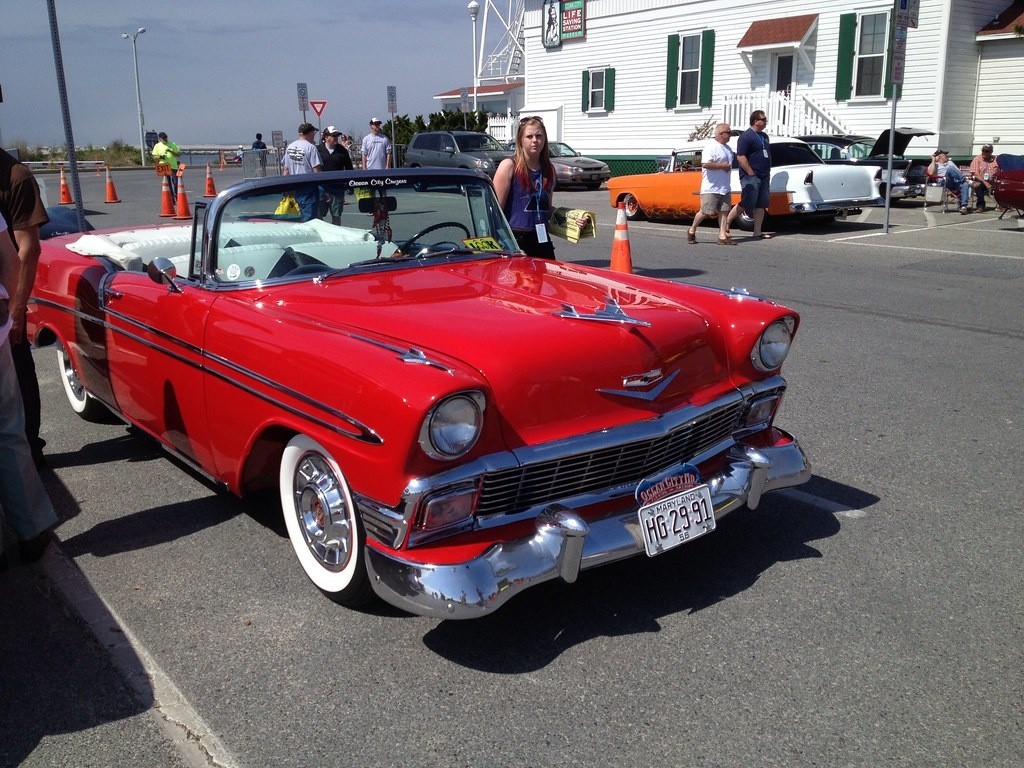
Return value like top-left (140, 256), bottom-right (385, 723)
top-left (975, 207), bottom-right (984, 213)
top-left (959, 207), bottom-right (968, 215)
top-left (716, 236), bottom-right (738, 245)
top-left (687, 227), bottom-right (697, 244)
top-left (753, 233), bottom-right (772, 239)
top-left (726, 230), bottom-right (732, 237)
top-left (995, 206), bottom-right (1003, 212)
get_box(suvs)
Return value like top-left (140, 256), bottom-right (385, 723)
top-left (404, 129), bottom-right (512, 198)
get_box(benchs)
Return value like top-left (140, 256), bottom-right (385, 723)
top-left (287, 240), bottom-right (399, 271)
top-left (169, 243), bottom-right (287, 282)
top-left (121, 228), bottom-right (322, 266)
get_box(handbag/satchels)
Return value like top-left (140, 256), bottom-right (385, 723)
top-left (274, 197), bottom-right (300, 214)
top-left (354, 187), bottom-right (371, 201)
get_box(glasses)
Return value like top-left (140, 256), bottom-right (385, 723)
top-left (520, 116), bottom-right (543, 123)
top-left (722, 131), bottom-right (732, 134)
top-left (757, 117), bottom-right (766, 122)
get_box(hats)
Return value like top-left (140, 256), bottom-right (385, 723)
top-left (980, 144), bottom-right (993, 152)
top-left (323, 126), bottom-right (342, 136)
top-left (238, 144), bottom-right (243, 148)
top-left (369, 117), bottom-right (383, 124)
top-left (159, 132), bottom-right (168, 138)
top-left (933, 149), bottom-right (949, 157)
top-left (297, 123), bottom-right (319, 135)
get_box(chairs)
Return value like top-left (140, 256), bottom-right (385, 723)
top-left (923, 168), bottom-right (1001, 214)
top-left (814, 149), bottom-right (822, 159)
top-left (829, 148), bottom-right (841, 159)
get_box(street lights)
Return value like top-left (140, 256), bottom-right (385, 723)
top-left (121, 26), bottom-right (149, 167)
top-left (467, 0), bottom-right (481, 124)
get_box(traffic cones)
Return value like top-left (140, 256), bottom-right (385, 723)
top-left (609, 201), bottom-right (635, 274)
top-left (95, 166), bottom-right (101, 176)
top-left (172, 174), bottom-right (193, 220)
top-left (203, 162), bottom-right (219, 197)
top-left (158, 173), bottom-right (177, 217)
top-left (57, 167), bottom-right (76, 205)
top-left (103, 166), bottom-right (121, 203)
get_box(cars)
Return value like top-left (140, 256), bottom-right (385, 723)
top-left (994, 169), bottom-right (1024, 212)
top-left (792, 126), bottom-right (933, 203)
top-left (17, 164), bottom-right (815, 620)
top-left (606, 135), bottom-right (883, 228)
top-left (506, 141), bottom-right (610, 191)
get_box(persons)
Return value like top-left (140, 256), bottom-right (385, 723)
top-left (0, 148), bottom-right (58, 566)
top-left (152, 131), bottom-right (181, 205)
top-left (725, 110), bottom-right (772, 240)
top-left (927, 149), bottom-right (981, 215)
top-left (283, 123), bottom-right (354, 226)
top-left (252, 133), bottom-right (267, 177)
top-left (493, 116), bottom-right (589, 260)
top-left (341, 133), bottom-right (359, 169)
top-left (361, 117), bottom-right (392, 215)
top-left (969, 145), bottom-right (1003, 213)
top-left (687, 124), bottom-right (737, 245)
